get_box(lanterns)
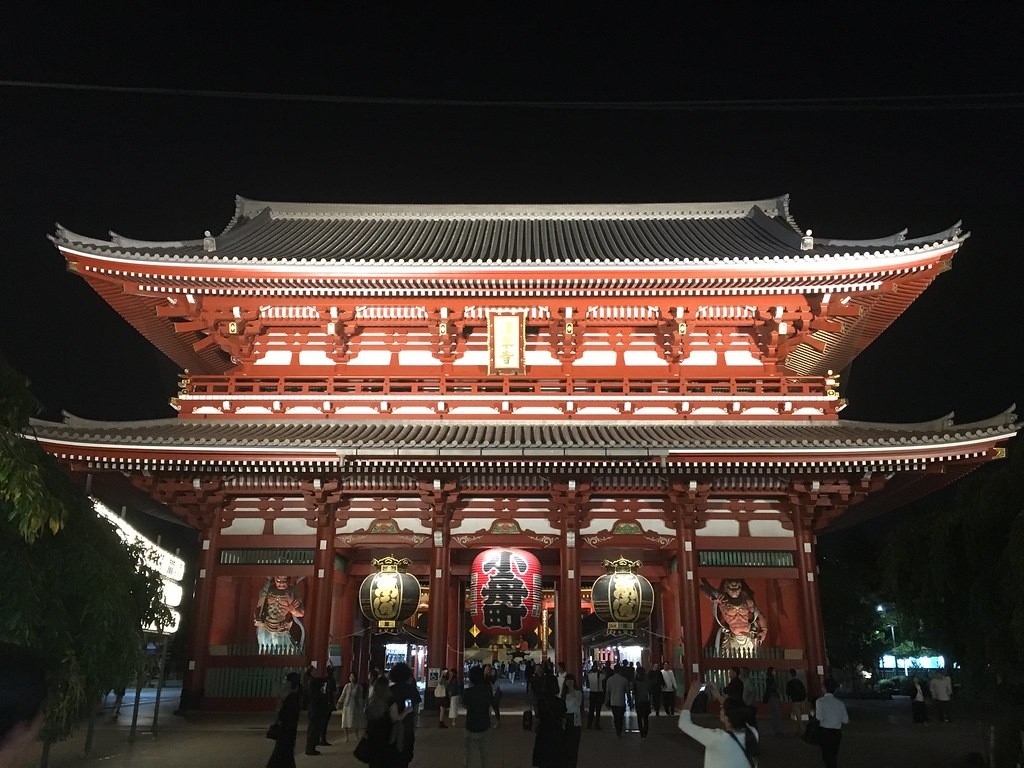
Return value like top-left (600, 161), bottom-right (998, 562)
top-left (470, 547), bottom-right (543, 644)
top-left (358, 557), bottom-right (422, 635)
top-left (591, 558), bottom-right (655, 636)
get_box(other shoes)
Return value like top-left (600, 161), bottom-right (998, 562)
top-left (306, 749), bottom-right (320, 755)
top-left (668, 713), bottom-right (675, 717)
top-left (452, 721), bottom-right (456, 726)
top-left (439, 723), bottom-right (448, 728)
top-left (641, 736), bottom-right (648, 741)
top-left (587, 725), bottom-right (603, 730)
top-left (343, 737), bottom-right (349, 742)
top-left (320, 741), bottom-right (332, 746)
top-left (617, 732), bottom-right (623, 738)
top-left (354, 739), bottom-right (359, 744)
top-left (493, 722), bottom-right (503, 729)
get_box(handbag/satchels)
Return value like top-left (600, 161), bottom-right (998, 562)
top-left (597, 692), bottom-right (605, 703)
top-left (434, 676), bottom-right (446, 698)
top-left (353, 736), bottom-right (371, 763)
top-left (802, 717), bottom-right (822, 745)
top-left (266, 718), bottom-right (283, 740)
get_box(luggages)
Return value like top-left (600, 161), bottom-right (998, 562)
top-left (523, 710), bottom-right (533, 731)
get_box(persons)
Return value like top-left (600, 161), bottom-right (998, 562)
top-left (911, 676), bottom-right (931, 728)
top-left (266, 662), bottom-right (422, 768)
top-left (698, 577), bottom-right (768, 659)
top-left (253, 576), bottom-right (305, 655)
top-left (679, 681), bottom-right (759, 768)
top-left (433, 659), bottom-right (583, 768)
top-left (930, 672), bottom-right (953, 722)
top-left (723, 665), bottom-right (850, 768)
top-left (584, 660), bottom-right (678, 742)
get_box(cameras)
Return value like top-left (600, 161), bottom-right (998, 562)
top-left (699, 684), bottom-right (707, 692)
top-left (405, 699), bottom-right (411, 708)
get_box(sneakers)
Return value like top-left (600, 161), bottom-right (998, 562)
top-left (915, 722), bottom-right (930, 727)
top-left (942, 720), bottom-right (957, 725)
